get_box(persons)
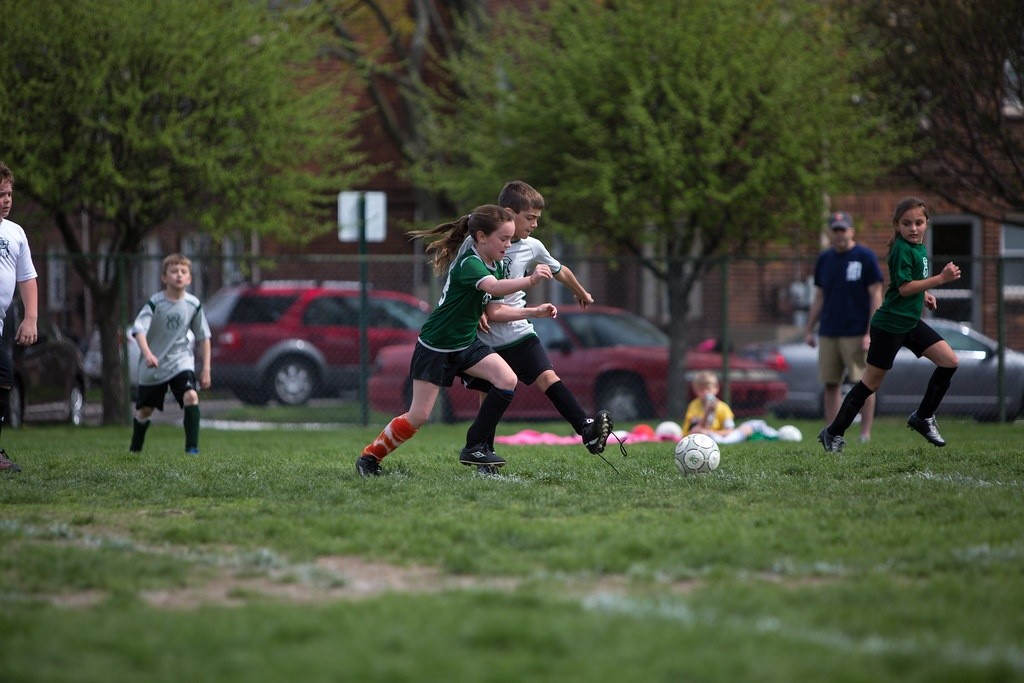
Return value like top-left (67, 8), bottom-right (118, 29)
top-left (807, 211), bottom-right (883, 444)
top-left (356, 205), bottom-right (557, 480)
top-left (682, 369), bottom-right (781, 444)
top-left (818, 198), bottom-right (961, 455)
top-left (0, 163), bottom-right (38, 472)
top-left (458, 181), bottom-right (613, 476)
top-left (129, 253), bottom-right (211, 462)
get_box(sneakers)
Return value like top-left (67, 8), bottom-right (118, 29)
top-left (581, 409), bottom-right (614, 455)
top-left (460, 441), bottom-right (507, 475)
top-left (817, 425), bottom-right (846, 452)
top-left (907, 409), bottom-right (946, 447)
top-left (356, 454), bottom-right (380, 477)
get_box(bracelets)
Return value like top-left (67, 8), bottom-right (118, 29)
top-left (530, 275), bottom-right (536, 287)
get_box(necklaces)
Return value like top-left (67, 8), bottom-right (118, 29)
top-left (475, 246), bottom-right (493, 264)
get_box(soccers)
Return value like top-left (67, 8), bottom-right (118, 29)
top-left (674, 433), bottom-right (720, 474)
top-left (777, 424), bottom-right (802, 442)
top-left (656, 421), bottom-right (683, 440)
top-left (630, 425), bottom-right (655, 438)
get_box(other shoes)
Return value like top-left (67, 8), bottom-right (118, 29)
top-left (0, 449), bottom-right (21, 472)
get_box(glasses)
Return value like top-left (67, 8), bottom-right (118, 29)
top-left (833, 228), bottom-right (845, 232)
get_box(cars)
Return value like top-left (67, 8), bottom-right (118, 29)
top-left (0, 295), bottom-right (91, 428)
top-left (368, 301), bottom-right (788, 423)
top-left (742, 317), bottom-right (1024, 421)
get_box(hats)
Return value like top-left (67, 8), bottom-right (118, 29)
top-left (829, 210), bottom-right (854, 230)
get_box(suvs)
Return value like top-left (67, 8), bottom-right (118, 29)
top-left (201, 275), bottom-right (440, 406)
